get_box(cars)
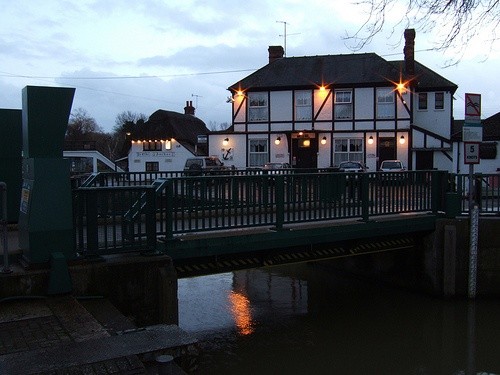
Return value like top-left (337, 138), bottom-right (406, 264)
top-left (377, 160), bottom-right (407, 185)
top-left (256, 162), bottom-right (295, 186)
top-left (335, 160), bottom-right (370, 185)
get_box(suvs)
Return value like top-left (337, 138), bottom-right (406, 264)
top-left (183, 156), bottom-right (231, 186)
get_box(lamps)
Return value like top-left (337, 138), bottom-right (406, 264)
top-left (321, 136), bottom-right (327, 144)
top-left (368, 135), bottom-right (374, 144)
top-left (274, 137), bottom-right (281, 145)
top-left (399, 135), bottom-right (406, 144)
top-left (223, 138), bottom-right (229, 145)
